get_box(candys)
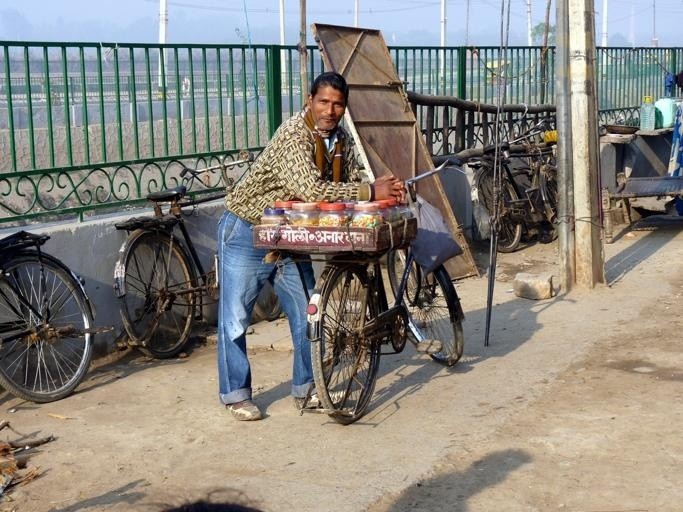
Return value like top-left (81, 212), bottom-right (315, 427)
top-left (290, 214), bottom-right (384, 228)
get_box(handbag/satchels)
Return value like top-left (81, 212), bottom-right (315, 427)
top-left (407, 198), bottom-right (463, 275)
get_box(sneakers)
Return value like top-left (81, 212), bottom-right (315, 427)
top-left (226, 400), bottom-right (262, 421)
top-left (294, 390), bottom-right (345, 409)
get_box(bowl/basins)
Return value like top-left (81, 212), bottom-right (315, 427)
top-left (603, 124), bottom-right (640, 134)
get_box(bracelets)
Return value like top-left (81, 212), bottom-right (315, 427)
top-left (368, 183), bottom-right (375, 202)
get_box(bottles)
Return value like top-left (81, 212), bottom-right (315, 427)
top-left (655, 96), bottom-right (677, 129)
top-left (260, 198), bottom-right (412, 226)
top-left (640, 97), bottom-right (655, 131)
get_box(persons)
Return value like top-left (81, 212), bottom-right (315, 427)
top-left (216, 72), bottom-right (407, 422)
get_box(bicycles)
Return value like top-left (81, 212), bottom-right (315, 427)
top-left (452, 106), bottom-right (579, 255)
top-left (111, 151), bottom-right (290, 361)
top-left (0, 228), bottom-right (118, 407)
top-left (288, 159), bottom-right (469, 419)
